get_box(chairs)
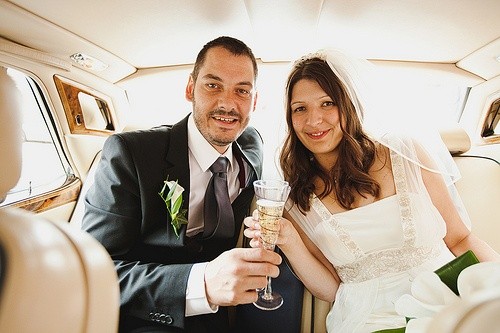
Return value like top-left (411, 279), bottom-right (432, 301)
top-left (0, 66), bottom-right (500, 333)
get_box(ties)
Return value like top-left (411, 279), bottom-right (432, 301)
top-left (199, 156), bottom-right (235, 253)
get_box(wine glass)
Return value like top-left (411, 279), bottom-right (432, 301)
top-left (254, 178), bottom-right (292, 311)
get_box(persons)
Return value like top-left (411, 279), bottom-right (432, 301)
top-left (81, 37), bottom-right (283, 333)
top-left (244, 51), bottom-right (500, 333)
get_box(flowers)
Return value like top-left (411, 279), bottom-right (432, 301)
top-left (159, 173), bottom-right (189, 240)
